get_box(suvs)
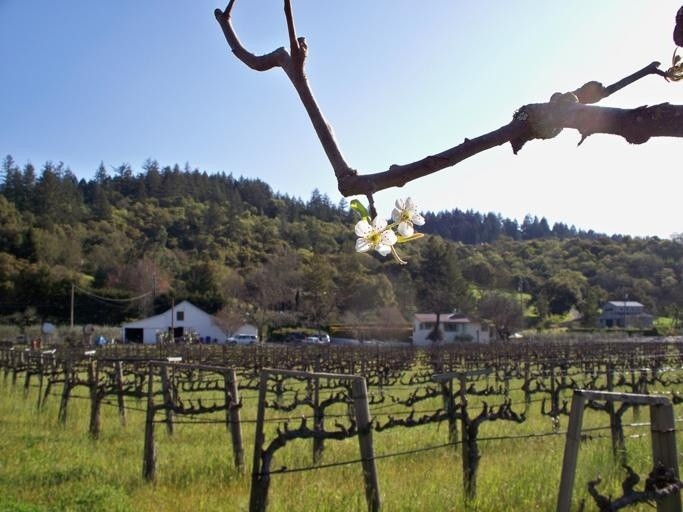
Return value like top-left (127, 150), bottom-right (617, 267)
top-left (225, 334), bottom-right (257, 346)
